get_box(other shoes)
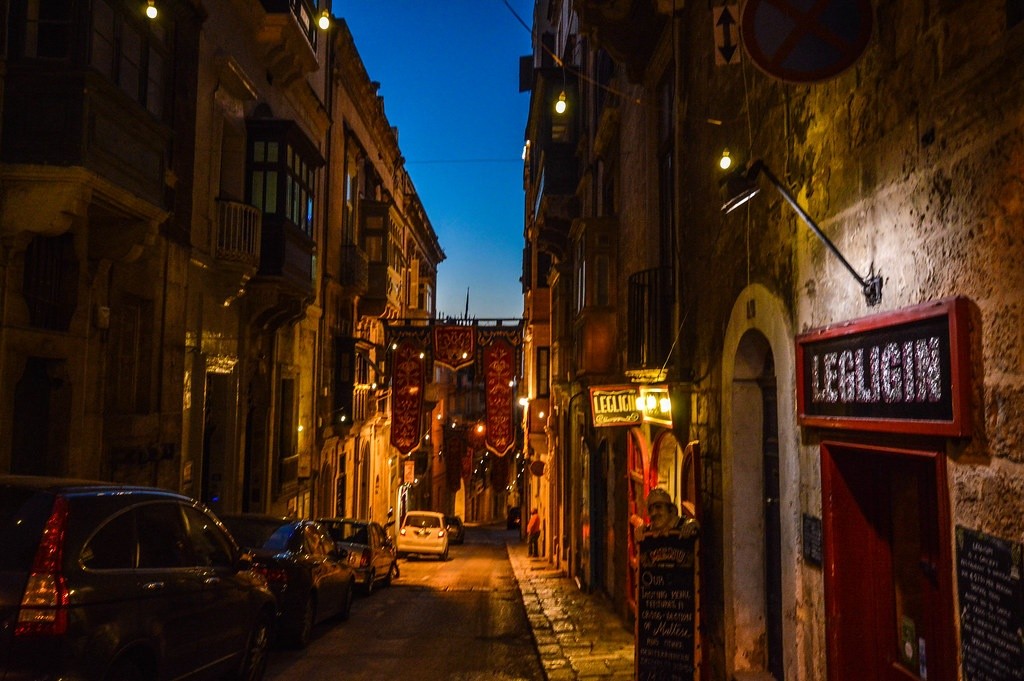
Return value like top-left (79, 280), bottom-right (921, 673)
top-left (528, 553), bottom-right (533, 558)
top-left (533, 554), bottom-right (539, 557)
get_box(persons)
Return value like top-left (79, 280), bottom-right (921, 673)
top-left (527, 507), bottom-right (541, 558)
top-left (634, 488), bottom-right (700, 542)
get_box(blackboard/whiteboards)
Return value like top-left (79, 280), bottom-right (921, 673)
top-left (634, 531), bottom-right (701, 681)
top-left (802, 512), bottom-right (822, 569)
top-left (954, 524), bottom-right (1024, 681)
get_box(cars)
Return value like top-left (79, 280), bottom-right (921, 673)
top-left (506, 508), bottom-right (521, 530)
top-left (443, 516), bottom-right (464, 544)
top-left (213, 511), bottom-right (355, 649)
top-left (313, 518), bottom-right (394, 594)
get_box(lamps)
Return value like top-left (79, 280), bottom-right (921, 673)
top-left (318, 407), bottom-right (347, 429)
top-left (717, 159), bottom-right (881, 305)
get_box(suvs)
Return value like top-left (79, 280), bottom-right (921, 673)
top-left (0, 473), bottom-right (278, 681)
top-left (396, 511), bottom-right (449, 561)
